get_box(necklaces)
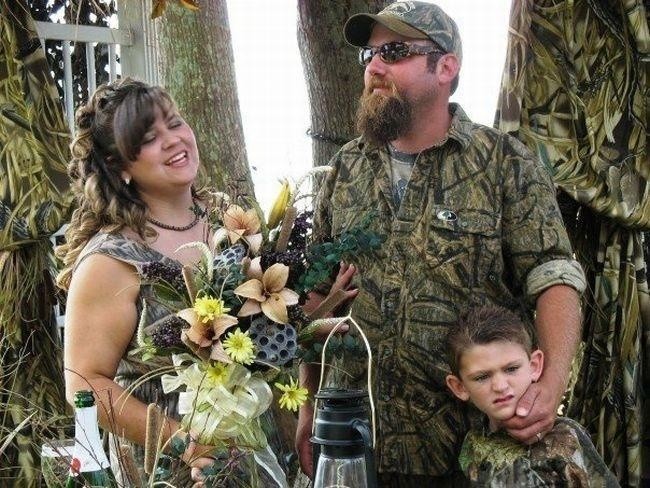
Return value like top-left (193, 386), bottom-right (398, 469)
top-left (145, 197), bottom-right (200, 231)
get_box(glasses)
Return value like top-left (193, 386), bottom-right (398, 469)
top-left (357, 40), bottom-right (443, 67)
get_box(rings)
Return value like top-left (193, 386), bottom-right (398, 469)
top-left (537, 433), bottom-right (542, 439)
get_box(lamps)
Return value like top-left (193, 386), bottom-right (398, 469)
top-left (309, 316), bottom-right (376, 488)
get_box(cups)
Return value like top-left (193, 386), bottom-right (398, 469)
top-left (40, 441), bottom-right (72, 487)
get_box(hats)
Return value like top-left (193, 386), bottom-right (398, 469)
top-left (344, 1), bottom-right (463, 64)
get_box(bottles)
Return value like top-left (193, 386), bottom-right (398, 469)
top-left (67, 391), bottom-right (117, 487)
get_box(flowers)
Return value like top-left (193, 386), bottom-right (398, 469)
top-left (129, 166), bottom-right (382, 488)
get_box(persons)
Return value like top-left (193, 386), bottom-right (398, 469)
top-left (444, 305), bottom-right (621, 488)
top-left (54, 76), bottom-right (357, 488)
top-left (293, 1), bottom-right (587, 488)
